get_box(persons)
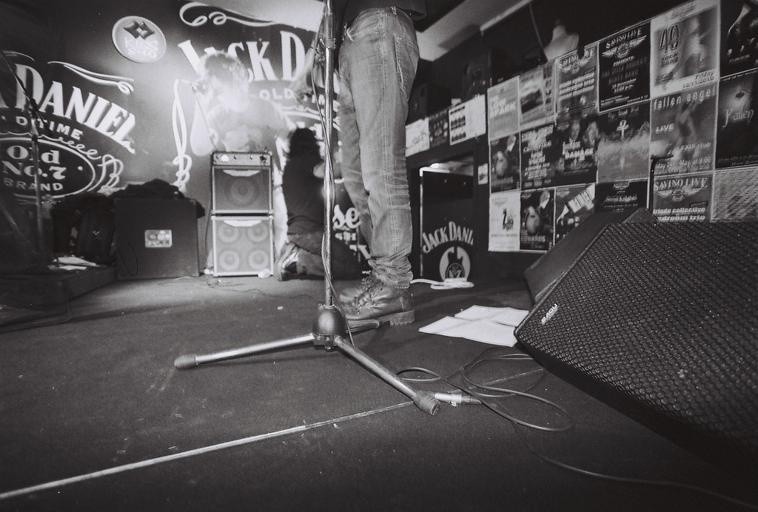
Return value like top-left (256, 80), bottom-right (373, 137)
top-left (662, 15), bottom-right (713, 91)
top-left (662, 98), bottom-right (697, 148)
top-left (491, 136), bottom-right (518, 193)
top-left (276, 126), bottom-right (364, 282)
top-left (520, 190), bottom-right (551, 249)
top-left (188, 52), bottom-right (296, 277)
top-left (553, 117), bottom-right (598, 177)
top-left (287, 0), bottom-right (431, 327)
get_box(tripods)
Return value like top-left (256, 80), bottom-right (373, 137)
top-left (4, 107), bottom-right (86, 322)
top-left (172, 0), bottom-right (442, 416)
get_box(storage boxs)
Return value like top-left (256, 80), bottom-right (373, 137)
top-left (114, 198), bottom-right (200, 281)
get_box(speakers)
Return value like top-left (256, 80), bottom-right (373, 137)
top-left (523, 207), bottom-right (660, 303)
top-left (211, 217), bottom-right (275, 277)
top-left (212, 166), bottom-right (272, 214)
top-left (513, 222), bottom-right (758, 452)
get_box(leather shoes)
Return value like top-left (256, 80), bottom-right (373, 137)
top-left (339, 274), bottom-right (415, 329)
top-left (273, 242), bottom-right (299, 282)
